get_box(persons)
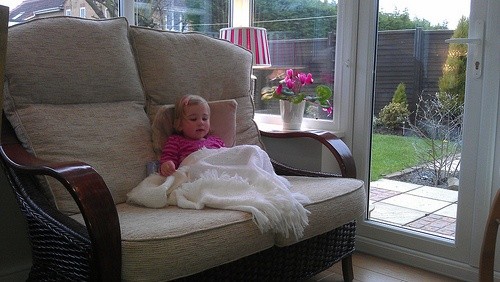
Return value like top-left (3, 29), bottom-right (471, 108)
top-left (160, 94), bottom-right (228, 177)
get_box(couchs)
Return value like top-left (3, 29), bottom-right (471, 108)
top-left (3, 15), bottom-right (368, 282)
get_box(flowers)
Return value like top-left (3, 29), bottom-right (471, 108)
top-left (261, 68), bottom-right (333, 116)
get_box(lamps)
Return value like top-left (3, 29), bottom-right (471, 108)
top-left (218, 26), bottom-right (272, 100)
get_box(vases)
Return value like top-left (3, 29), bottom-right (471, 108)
top-left (279, 99), bottom-right (306, 131)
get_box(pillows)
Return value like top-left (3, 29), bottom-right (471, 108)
top-left (150, 102), bottom-right (236, 165)
